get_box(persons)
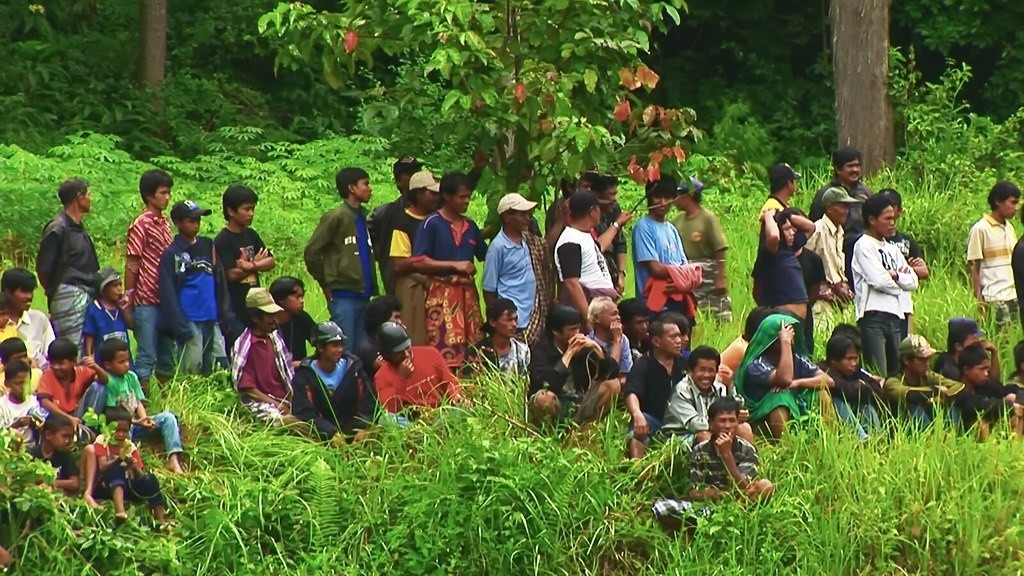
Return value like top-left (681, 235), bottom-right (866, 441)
top-left (0, 148), bottom-right (1024, 535)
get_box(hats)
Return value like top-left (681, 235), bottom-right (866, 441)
top-left (409, 171), bottom-right (440, 193)
top-left (678, 176), bottom-right (705, 195)
top-left (821, 186), bottom-right (862, 206)
top-left (570, 191), bottom-right (610, 212)
top-left (496, 192), bottom-right (539, 215)
top-left (94, 267), bottom-right (119, 293)
top-left (392, 154), bottom-right (426, 176)
top-left (879, 188), bottom-right (902, 206)
top-left (899, 335), bottom-right (937, 359)
top-left (948, 318), bottom-right (987, 337)
top-left (245, 287), bottom-right (285, 314)
top-left (170, 199), bottom-right (212, 219)
top-left (771, 163), bottom-right (802, 182)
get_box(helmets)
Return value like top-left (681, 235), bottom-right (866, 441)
top-left (377, 321), bottom-right (412, 355)
top-left (310, 320), bottom-right (348, 346)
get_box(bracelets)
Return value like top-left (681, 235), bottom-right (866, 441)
top-left (251, 261), bottom-right (256, 271)
top-left (618, 270), bottom-right (627, 277)
top-left (611, 221), bottom-right (621, 231)
